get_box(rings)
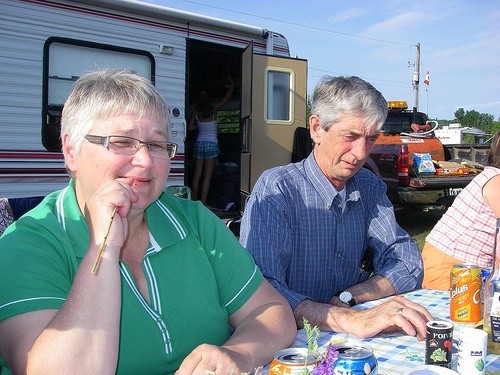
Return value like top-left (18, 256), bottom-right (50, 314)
top-left (396, 307), bottom-right (403, 315)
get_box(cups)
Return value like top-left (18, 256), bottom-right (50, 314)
top-left (405, 364), bottom-right (461, 375)
top-left (456, 327), bottom-right (488, 375)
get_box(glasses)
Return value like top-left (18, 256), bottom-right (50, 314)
top-left (85, 135), bottom-right (178, 159)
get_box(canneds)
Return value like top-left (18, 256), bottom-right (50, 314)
top-left (268, 340), bottom-right (379, 375)
top-left (425, 321), bottom-right (454, 370)
top-left (481, 267), bottom-right (500, 304)
top-left (450, 264), bottom-right (481, 323)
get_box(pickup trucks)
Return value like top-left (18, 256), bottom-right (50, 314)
top-left (362, 100), bottom-right (492, 216)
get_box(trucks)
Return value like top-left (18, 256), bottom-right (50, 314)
top-left (0, 0), bottom-right (308, 246)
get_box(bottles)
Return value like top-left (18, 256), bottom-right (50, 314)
top-left (483, 217), bottom-right (500, 354)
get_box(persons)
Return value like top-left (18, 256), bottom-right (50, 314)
top-left (421, 130), bottom-right (500, 291)
top-left (0, 69), bottom-right (297, 375)
top-left (239, 74), bottom-right (434, 339)
top-left (189, 106), bottom-right (221, 205)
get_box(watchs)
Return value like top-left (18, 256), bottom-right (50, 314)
top-left (335, 291), bottom-right (357, 307)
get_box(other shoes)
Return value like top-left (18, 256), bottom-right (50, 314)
top-left (217, 202), bottom-right (236, 211)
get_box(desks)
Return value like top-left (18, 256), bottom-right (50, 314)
top-left (245, 289), bottom-right (500, 375)
